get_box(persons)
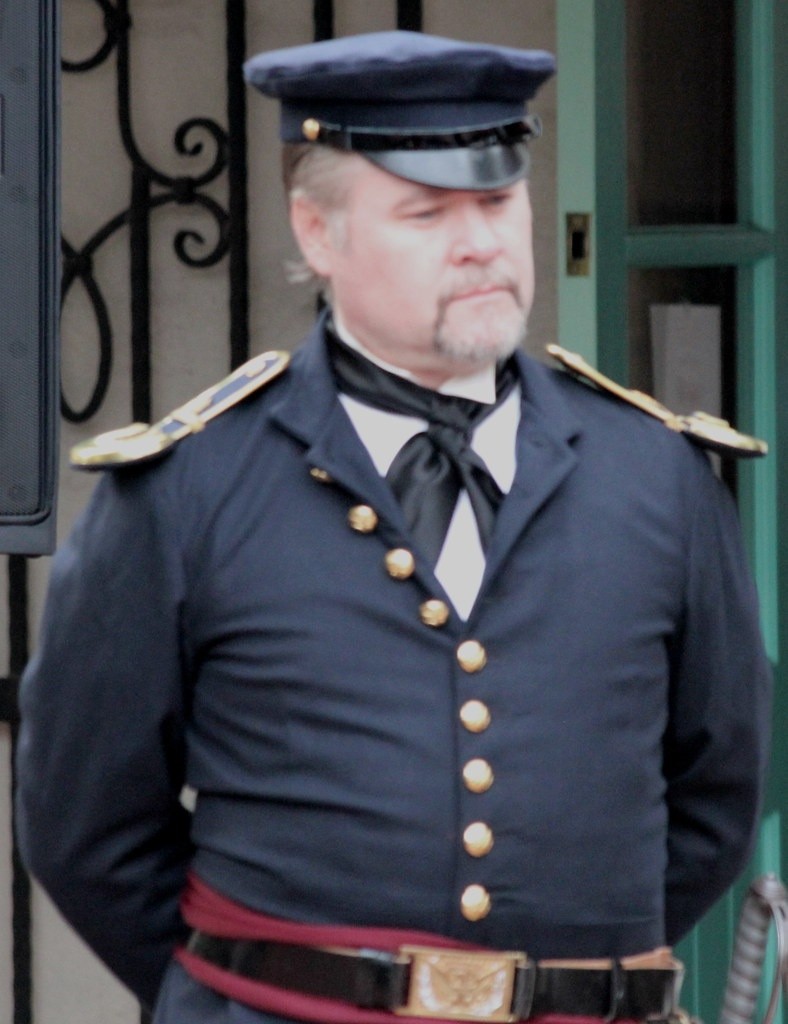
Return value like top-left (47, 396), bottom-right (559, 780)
top-left (15, 33), bottom-right (770, 1023)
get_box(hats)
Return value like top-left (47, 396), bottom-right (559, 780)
top-left (243, 30), bottom-right (558, 190)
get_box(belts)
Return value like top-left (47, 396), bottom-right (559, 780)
top-left (178, 914), bottom-right (689, 1022)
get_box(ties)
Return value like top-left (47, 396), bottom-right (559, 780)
top-left (327, 335), bottom-right (525, 570)
